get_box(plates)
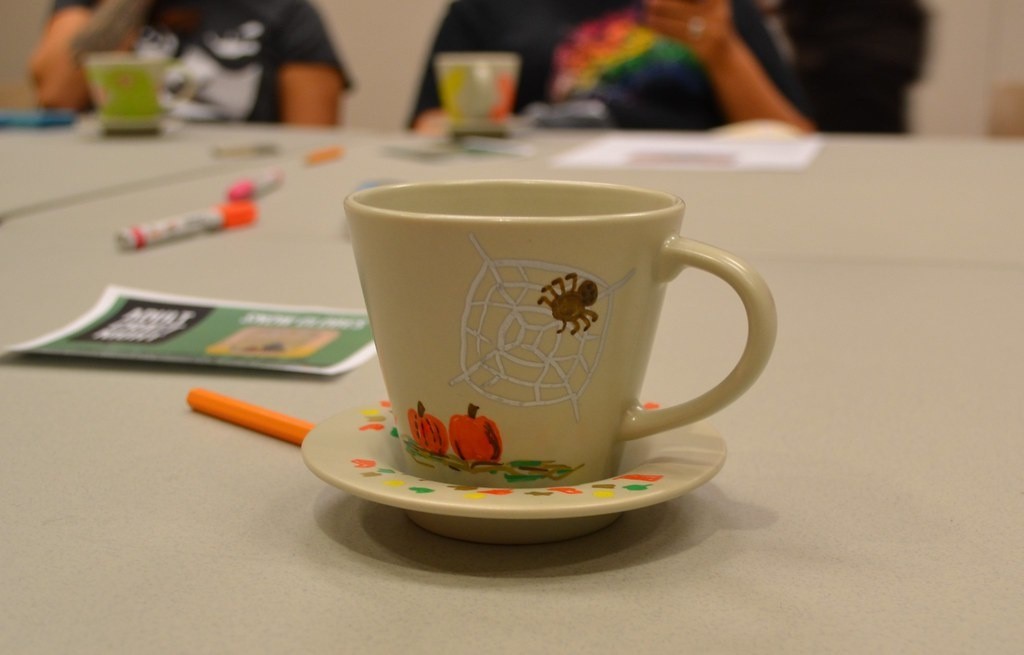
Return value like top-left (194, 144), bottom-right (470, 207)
top-left (300, 407), bottom-right (730, 546)
top-left (97, 109), bottom-right (172, 131)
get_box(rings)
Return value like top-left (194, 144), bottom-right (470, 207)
top-left (687, 19), bottom-right (703, 39)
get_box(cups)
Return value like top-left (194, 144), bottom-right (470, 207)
top-left (85, 61), bottom-right (194, 113)
top-left (434, 55), bottom-right (519, 122)
top-left (345, 182), bottom-right (777, 486)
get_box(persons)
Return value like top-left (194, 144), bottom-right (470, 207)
top-left (28, 0), bottom-right (353, 126)
top-left (407, 0), bottom-right (925, 138)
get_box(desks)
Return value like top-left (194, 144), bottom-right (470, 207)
top-left (0, 116), bottom-right (1024, 652)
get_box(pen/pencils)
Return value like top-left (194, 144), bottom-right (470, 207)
top-left (186, 388), bottom-right (316, 446)
top-left (303, 147), bottom-right (346, 167)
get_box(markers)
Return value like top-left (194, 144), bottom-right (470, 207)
top-left (115, 203), bottom-right (258, 249)
top-left (227, 167), bottom-right (282, 200)
